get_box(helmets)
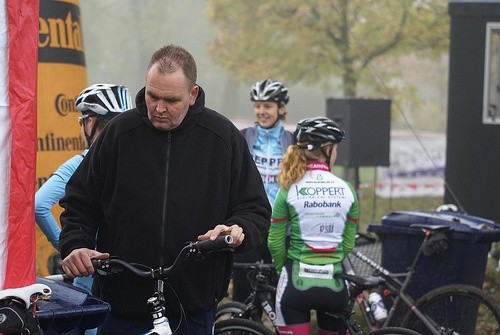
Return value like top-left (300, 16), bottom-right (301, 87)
top-left (74, 84), bottom-right (133, 116)
top-left (294, 117), bottom-right (345, 147)
top-left (250, 78), bottom-right (289, 105)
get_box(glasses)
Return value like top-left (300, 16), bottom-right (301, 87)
top-left (78, 113), bottom-right (95, 126)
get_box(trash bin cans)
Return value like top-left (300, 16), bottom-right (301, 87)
top-left (367, 209), bottom-right (500, 335)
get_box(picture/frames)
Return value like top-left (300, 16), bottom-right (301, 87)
top-left (482, 22), bottom-right (500, 125)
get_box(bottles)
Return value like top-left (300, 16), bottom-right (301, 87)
top-left (368, 292), bottom-right (388, 322)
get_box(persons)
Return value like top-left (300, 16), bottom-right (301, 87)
top-left (34, 84), bottom-right (136, 335)
top-left (59, 46), bottom-right (273, 335)
top-left (267, 117), bottom-right (358, 335)
top-left (234, 78), bottom-right (293, 314)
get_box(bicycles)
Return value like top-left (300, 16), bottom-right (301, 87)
top-left (215, 229), bottom-right (500, 335)
top-left (0, 234), bottom-right (234, 335)
